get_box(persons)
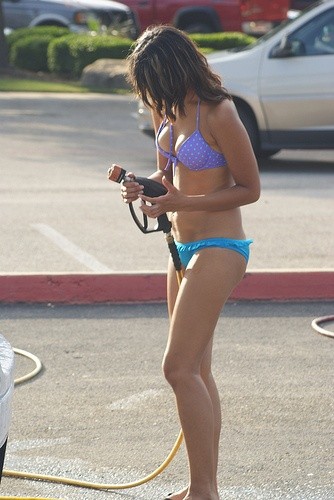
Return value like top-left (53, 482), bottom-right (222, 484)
top-left (120, 23), bottom-right (262, 499)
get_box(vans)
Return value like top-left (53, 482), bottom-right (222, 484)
top-left (124, 0), bottom-right (289, 37)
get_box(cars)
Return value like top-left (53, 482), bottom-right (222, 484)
top-left (1, 0), bottom-right (136, 41)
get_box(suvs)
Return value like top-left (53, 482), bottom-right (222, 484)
top-left (131, 2), bottom-right (334, 165)
